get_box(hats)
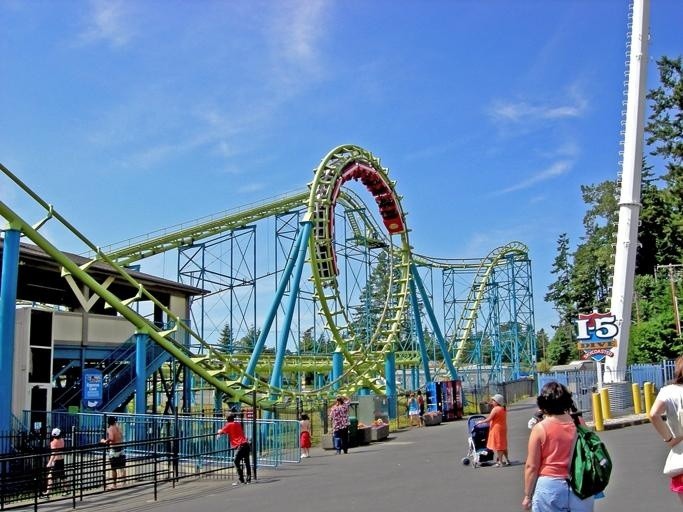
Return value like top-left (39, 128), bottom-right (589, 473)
top-left (492, 393), bottom-right (504, 405)
top-left (52, 428), bottom-right (61, 436)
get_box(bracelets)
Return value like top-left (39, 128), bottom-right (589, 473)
top-left (663, 436), bottom-right (672, 442)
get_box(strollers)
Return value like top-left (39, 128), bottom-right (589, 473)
top-left (461, 414), bottom-right (494, 469)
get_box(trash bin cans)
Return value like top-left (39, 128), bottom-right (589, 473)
top-left (345, 416), bottom-right (359, 448)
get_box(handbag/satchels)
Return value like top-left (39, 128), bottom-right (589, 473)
top-left (570, 414), bottom-right (612, 500)
top-left (663, 410), bottom-right (683, 477)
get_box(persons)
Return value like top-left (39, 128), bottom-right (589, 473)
top-left (475, 393), bottom-right (511, 468)
top-left (648, 357), bottom-right (682, 506)
top-left (520, 381), bottom-right (605, 512)
top-left (298, 412), bottom-right (312, 459)
top-left (98, 415), bottom-right (127, 491)
top-left (405, 391), bottom-right (422, 428)
top-left (214, 412), bottom-right (252, 486)
top-left (325, 394), bottom-right (354, 455)
top-left (415, 389), bottom-right (427, 426)
top-left (41, 427), bottom-right (71, 499)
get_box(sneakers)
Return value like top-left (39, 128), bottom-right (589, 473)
top-left (493, 461), bottom-right (510, 467)
top-left (301, 454), bottom-right (309, 458)
